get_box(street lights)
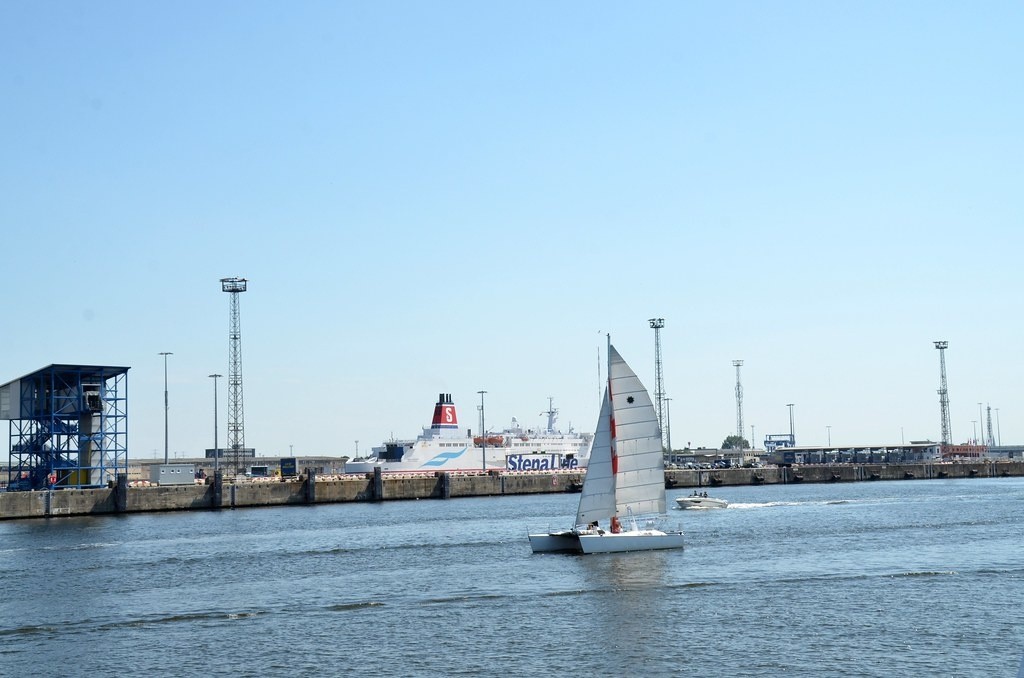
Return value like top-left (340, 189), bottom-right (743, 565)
top-left (476, 406), bottom-right (483, 435)
top-left (663, 398), bottom-right (673, 462)
top-left (158, 351), bottom-right (173, 466)
top-left (208, 374), bottom-right (224, 471)
top-left (750, 425), bottom-right (756, 450)
top-left (978, 403), bottom-right (983, 444)
top-left (994, 409), bottom-right (1000, 446)
top-left (477, 391), bottom-right (488, 472)
top-left (825, 426), bottom-right (831, 446)
top-left (786, 403), bottom-right (795, 447)
top-left (972, 420), bottom-right (977, 445)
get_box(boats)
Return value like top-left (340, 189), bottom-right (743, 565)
top-left (486, 396), bottom-right (594, 458)
top-left (675, 491), bottom-right (728, 509)
top-left (320, 392), bottom-right (595, 472)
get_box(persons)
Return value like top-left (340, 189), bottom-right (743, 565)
top-left (693, 490), bottom-right (698, 497)
top-left (699, 491), bottom-right (708, 497)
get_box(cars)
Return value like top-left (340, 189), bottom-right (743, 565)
top-left (663, 460), bottom-right (726, 470)
top-left (746, 463), bottom-right (763, 468)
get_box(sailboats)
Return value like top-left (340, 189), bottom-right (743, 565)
top-left (528, 386), bottom-right (614, 551)
top-left (579, 331), bottom-right (685, 552)
